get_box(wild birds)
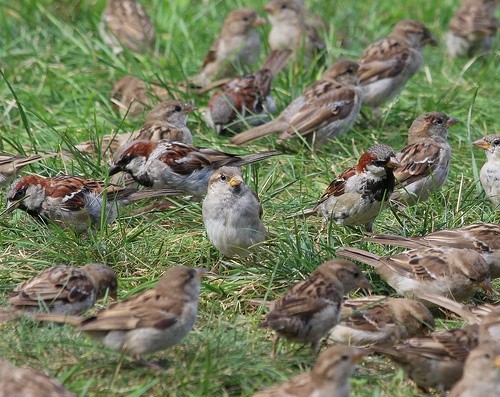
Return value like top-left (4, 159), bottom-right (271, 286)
top-left (321, 295), bottom-right (434, 355)
top-left (443, 0), bottom-right (500, 59)
top-left (0, 361), bottom-right (75, 397)
top-left (355, 19), bottom-right (439, 121)
top-left (0, 264), bottom-right (117, 329)
top-left (203, 165), bottom-right (267, 265)
top-left (35, 266), bottom-right (209, 376)
top-left (104, 100), bottom-right (198, 195)
top-left (102, 0), bottom-right (173, 69)
top-left (0, 150), bottom-right (58, 182)
top-left (262, 259), bottom-right (373, 360)
top-left (42, 0), bottom-right (368, 166)
top-left (284, 144), bottom-right (401, 240)
top-left (231, 59), bottom-right (393, 152)
top-left (386, 112), bottom-right (460, 211)
top-left (7, 175), bottom-right (184, 241)
top-left (333, 132), bottom-right (500, 396)
top-left (247, 345), bottom-right (368, 397)
top-left (109, 142), bottom-right (284, 208)
